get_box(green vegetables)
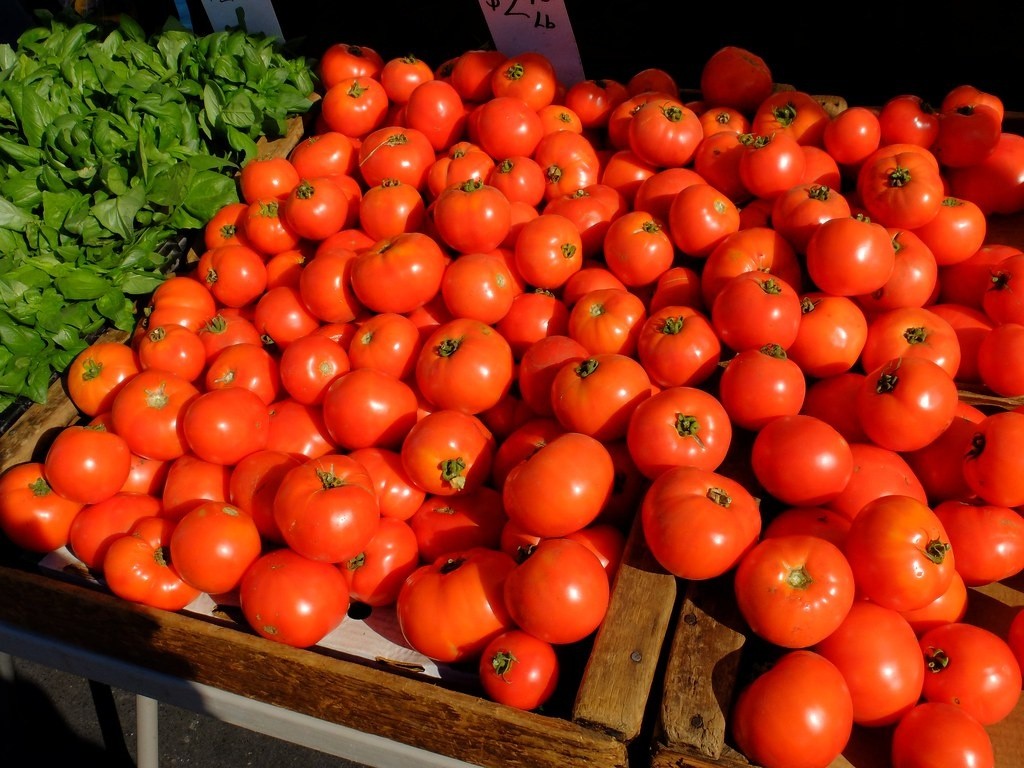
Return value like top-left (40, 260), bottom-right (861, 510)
top-left (0, 17), bottom-right (318, 415)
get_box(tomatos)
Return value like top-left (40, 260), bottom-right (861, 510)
top-left (0, 45), bottom-right (1024, 768)
